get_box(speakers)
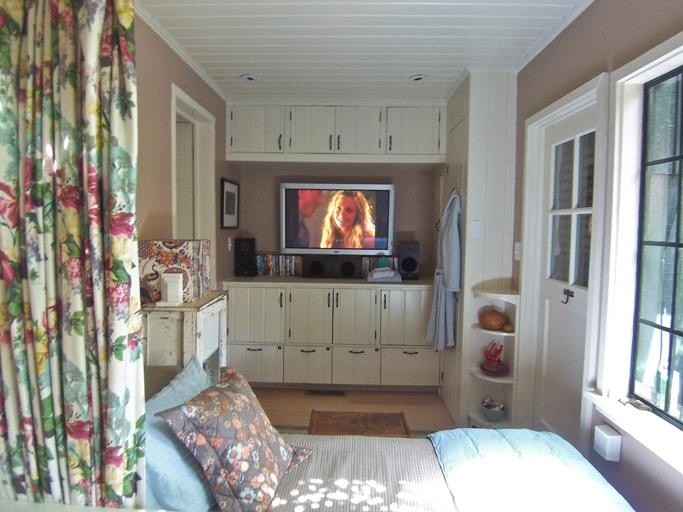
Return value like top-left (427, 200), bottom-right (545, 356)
top-left (235, 237), bottom-right (257, 277)
top-left (302, 255), bottom-right (362, 279)
top-left (399, 241), bottom-right (420, 279)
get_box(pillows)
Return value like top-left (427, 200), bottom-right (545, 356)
top-left (153, 367), bottom-right (312, 512)
top-left (145, 352), bottom-right (217, 512)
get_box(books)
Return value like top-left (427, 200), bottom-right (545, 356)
top-left (362, 257), bottom-right (398, 278)
top-left (256, 255), bottom-right (302, 276)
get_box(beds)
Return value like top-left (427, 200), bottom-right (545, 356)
top-left (145, 426), bottom-right (638, 512)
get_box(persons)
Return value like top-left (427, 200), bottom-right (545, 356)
top-left (298, 190), bottom-right (324, 246)
top-left (319, 190), bottom-right (375, 248)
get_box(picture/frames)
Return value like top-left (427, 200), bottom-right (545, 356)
top-left (220, 176), bottom-right (240, 230)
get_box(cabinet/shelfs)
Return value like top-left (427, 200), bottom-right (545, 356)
top-left (224, 101), bottom-right (447, 164)
top-left (141, 289), bottom-right (228, 385)
top-left (469, 287), bottom-right (520, 428)
top-left (433, 66), bottom-right (516, 429)
top-left (222, 276), bottom-right (441, 397)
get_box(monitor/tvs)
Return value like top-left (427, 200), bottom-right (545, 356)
top-left (279, 181), bottom-right (395, 256)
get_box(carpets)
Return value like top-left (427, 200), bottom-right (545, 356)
top-left (308, 409), bottom-right (411, 437)
